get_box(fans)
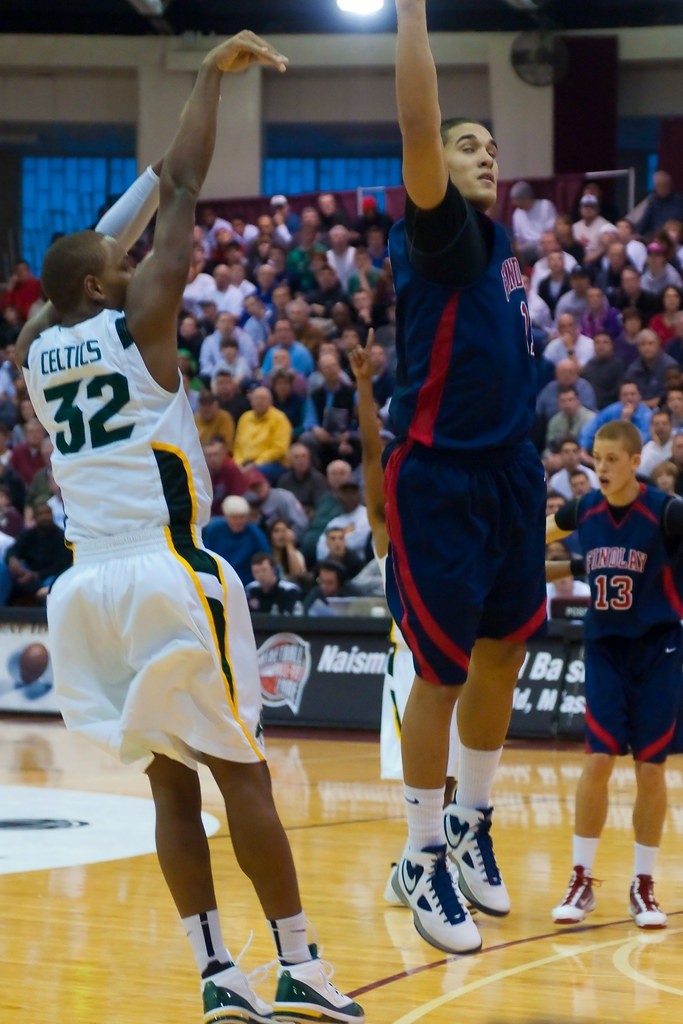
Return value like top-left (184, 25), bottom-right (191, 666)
top-left (510, 24), bottom-right (571, 86)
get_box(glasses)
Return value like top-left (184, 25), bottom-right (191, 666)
top-left (316, 578), bottom-right (335, 584)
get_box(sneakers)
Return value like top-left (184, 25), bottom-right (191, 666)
top-left (383, 842), bottom-right (482, 954)
top-left (628, 874), bottom-right (668, 928)
top-left (443, 803), bottom-right (510, 917)
top-left (200, 929), bottom-right (296, 1024)
top-left (274, 943), bottom-right (365, 1024)
top-left (551, 865), bottom-right (595, 924)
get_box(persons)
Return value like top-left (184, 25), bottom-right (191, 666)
top-left (544, 416), bottom-right (683, 931)
top-left (181, 191), bottom-right (395, 615)
top-left (16, 25), bottom-right (370, 1023)
top-left (503, 163), bottom-right (683, 597)
top-left (368, 1), bottom-right (552, 958)
top-left (0, 259), bottom-right (73, 610)
top-left (348, 338), bottom-right (468, 901)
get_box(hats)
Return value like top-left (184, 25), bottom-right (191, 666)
top-left (270, 194), bottom-right (289, 204)
top-left (579, 195), bottom-right (598, 206)
top-left (242, 471), bottom-right (264, 485)
top-left (569, 264), bottom-right (588, 276)
top-left (222, 497), bottom-right (249, 515)
top-left (337, 480), bottom-right (360, 492)
top-left (363, 197), bottom-right (376, 210)
top-left (508, 181), bottom-right (533, 197)
